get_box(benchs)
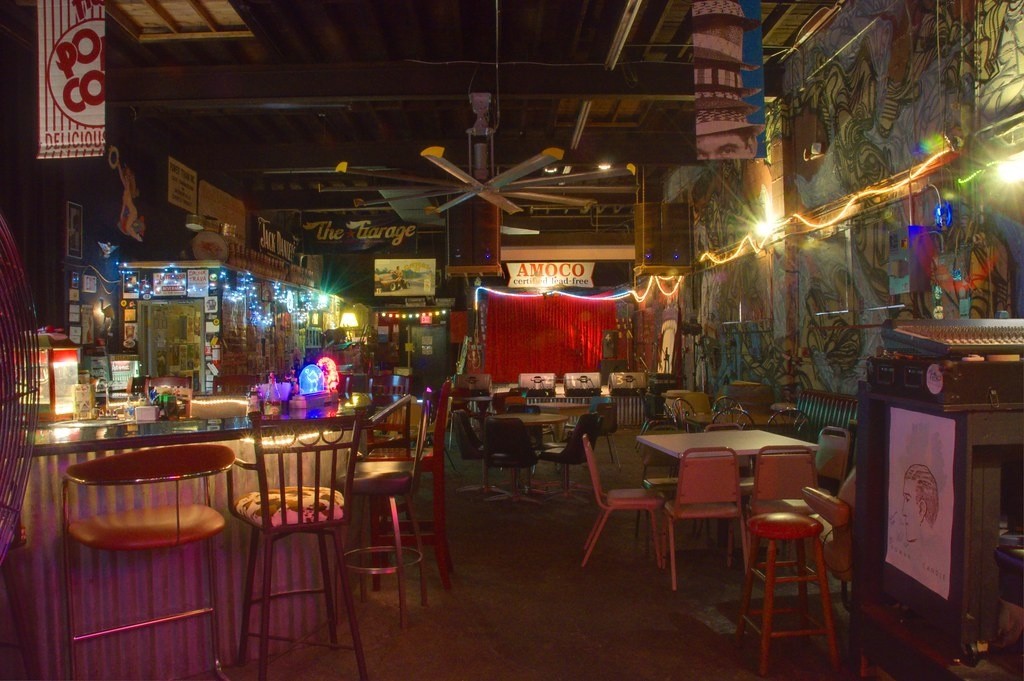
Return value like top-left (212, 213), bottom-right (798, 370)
top-left (793, 390), bottom-right (859, 445)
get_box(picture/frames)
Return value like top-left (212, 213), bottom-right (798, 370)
top-left (65, 200), bottom-right (83, 260)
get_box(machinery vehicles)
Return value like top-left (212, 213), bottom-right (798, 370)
top-left (378, 272), bottom-right (410, 291)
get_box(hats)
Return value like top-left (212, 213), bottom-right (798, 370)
top-left (692, 0), bottom-right (765, 136)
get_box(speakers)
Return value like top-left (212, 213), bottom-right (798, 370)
top-left (455, 373), bottom-right (494, 398)
top-left (448, 194), bottom-right (498, 267)
top-left (591, 261), bottom-right (634, 289)
top-left (466, 263), bottom-right (511, 288)
top-left (518, 373), bottom-right (557, 397)
top-left (634, 202), bottom-right (691, 267)
top-left (608, 372), bottom-right (647, 396)
top-left (563, 372), bottom-right (601, 397)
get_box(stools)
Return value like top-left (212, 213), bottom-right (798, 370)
top-left (335, 386), bottom-right (432, 631)
top-left (365, 393), bottom-right (420, 569)
top-left (63, 445), bottom-right (231, 681)
top-left (366, 379), bottom-right (452, 593)
top-left (0, 480), bottom-right (37, 681)
top-left (226, 412), bottom-right (368, 681)
top-left (734, 512), bottom-right (843, 675)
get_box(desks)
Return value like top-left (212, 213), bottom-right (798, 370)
top-left (484, 414), bottom-right (568, 497)
top-left (685, 414), bottom-right (804, 430)
top-left (635, 430), bottom-right (819, 560)
top-left (531, 402), bottom-right (592, 440)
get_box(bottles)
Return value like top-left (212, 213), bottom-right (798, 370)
top-left (263, 378), bottom-right (282, 420)
top-left (246, 387), bottom-right (259, 422)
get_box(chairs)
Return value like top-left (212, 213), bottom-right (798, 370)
top-left (483, 417), bottom-right (543, 505)
top-left (671, 397), bottom-right (696, 425)
top-left (594, 403), bottom-right (621, 469)
top-left (816, 426), bottom-right (853, 481)
top-left (541, 415), bottom-right (604, 504)
top-left (662, 447), bottom-right (750, 591)
top-left (451, 409), bottom-right (511, 496)
top-left (801, 465), bottom-right (856, 592)
top-left (746, 445), bottom-right (819, 568)
top-left (767, 409), bottom-right (813, 443)
top-left (634, 388), bottom-right (676, 453)
top-left (582, 433), bottom-right (664, 571)
top-left (634, 425), bottom-right (697, 538)
top-left (701, 423), bottom-right (743, 539)
top-left (711, 395), bottom-right (743, 415)
top-left (712, 407), bottom-right (756, 430)
